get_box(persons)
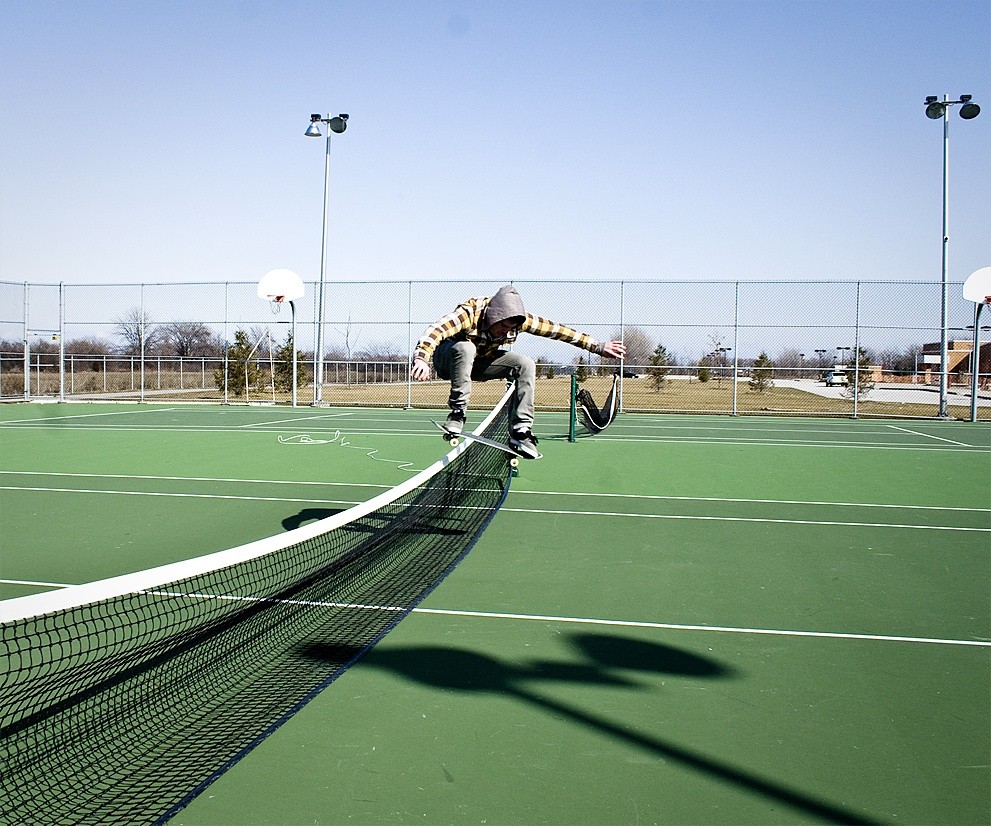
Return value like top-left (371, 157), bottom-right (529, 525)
top-left (410, 286), bottom-right (626, 457)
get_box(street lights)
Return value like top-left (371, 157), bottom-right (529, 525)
top-left (800, 353), bottom-right (805, 378)
top-left (706, 347), bottom-right (731, 378)
top-left (815, 350), bottom-right (826, 368)
top-left (922, 93), bottom-right (982, 421)
top-left (303, 112), bottom-right (351, 407)
top-left (837, 346), bottom-right (851, 368)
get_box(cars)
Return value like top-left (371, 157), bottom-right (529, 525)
top-left (825, 372), bottom-right (849, 387)
top-left (613, 368), bottom-right (639, 379)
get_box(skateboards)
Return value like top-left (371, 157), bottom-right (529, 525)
top-left (428, 417), bottom-right (544, 467)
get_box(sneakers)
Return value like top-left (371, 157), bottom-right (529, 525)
top-left (443, 409), bottom-right (464, 434)
top-left (510, 431), bottom-right (539, 458)
